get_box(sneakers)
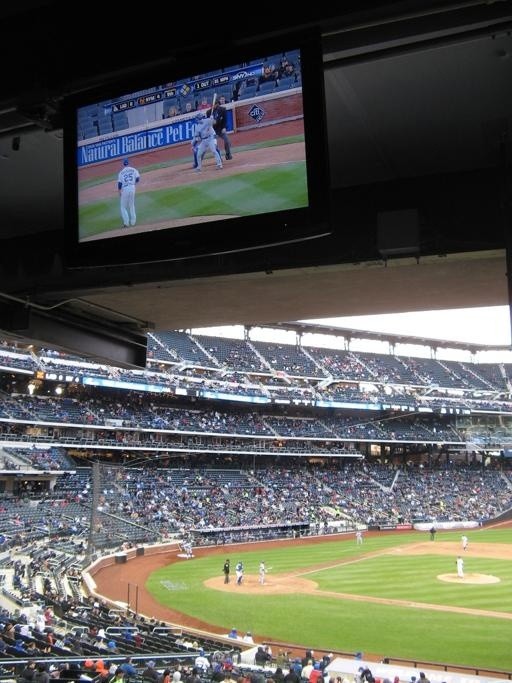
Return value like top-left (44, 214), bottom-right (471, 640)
top-left (192, 165), bottom-right (223, 173)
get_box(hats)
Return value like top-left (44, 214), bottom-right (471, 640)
top-left (85, 659), bottom-right (108, 677)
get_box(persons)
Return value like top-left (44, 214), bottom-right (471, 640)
top-left (265, 55), bottom-right (301, 80)
top-left (168, 94), bottom-right (234, 174)
top-left (118, 158), bottom-right (140, 228)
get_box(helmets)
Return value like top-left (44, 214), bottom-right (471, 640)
top-left (122, 158), bottom-right (129, 165)
top-left (196, 113), bottom-right (203, 120)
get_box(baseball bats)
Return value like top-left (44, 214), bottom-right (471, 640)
top-left (211, 92), bottom-right (217, 115)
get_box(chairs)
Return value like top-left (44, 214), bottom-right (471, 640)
top-left (0, 612), bottom-right (242, 683)
